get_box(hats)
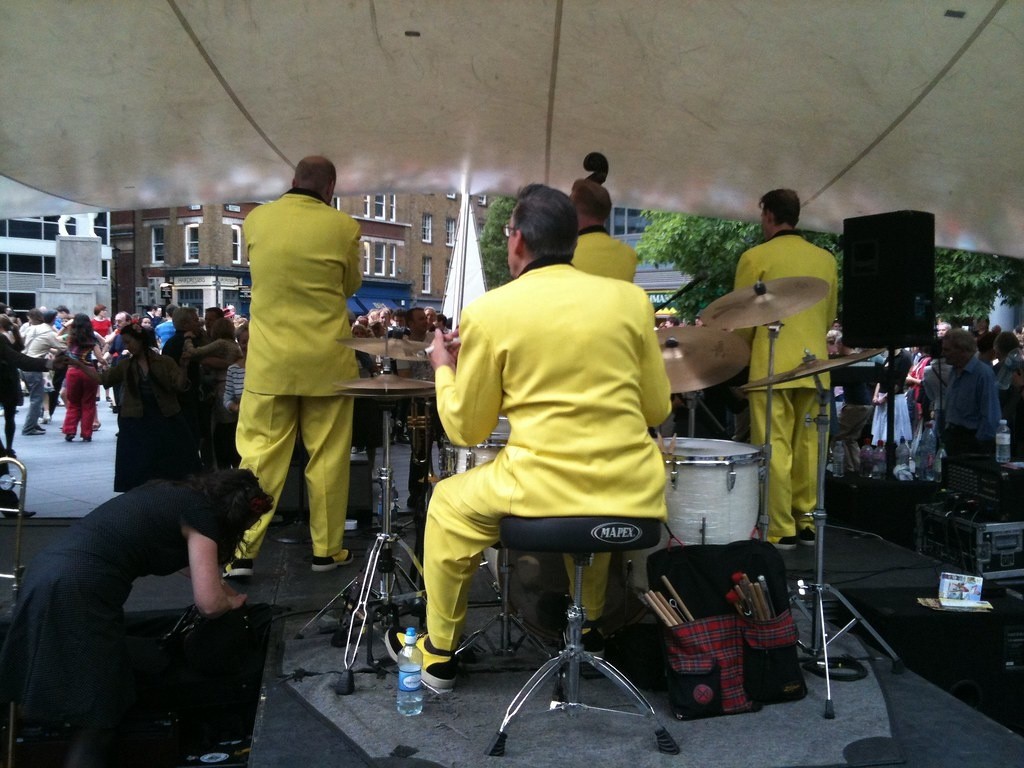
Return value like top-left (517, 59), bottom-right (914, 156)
top-left (42, 309), bottom-right (57, 319)
top-left (53, 305), bottom-right (70, 314)
top-left (222, 308), bottom-right (235, 318)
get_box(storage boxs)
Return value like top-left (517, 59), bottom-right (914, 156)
top-left (916, 502), bottom-right (1024, 580)
top-left (272, 443), bottom-right (372, 517)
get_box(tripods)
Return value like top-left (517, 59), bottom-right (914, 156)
top-left (453, 547), bottom-right (565, 673)
top-left (788, 373), bottom-right (905, 719)
top-left (292, 359), bottom-right (489, 695)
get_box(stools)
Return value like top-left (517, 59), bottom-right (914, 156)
top-left (460, 514), bottom-right (684, 754)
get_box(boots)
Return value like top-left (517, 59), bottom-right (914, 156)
top-left (38, 410), bottom-right (50, 424)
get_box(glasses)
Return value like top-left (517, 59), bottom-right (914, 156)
top-left (114, 319), bottom-right (126, 325)
top-left (501, 223), bottom-right (514, 238)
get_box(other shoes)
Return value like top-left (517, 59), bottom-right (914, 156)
top-left (96, 397), bottom-right (101, 403)
top-left (112, 407), bottom-right (118, 413)
top-left (56, 400), bottom-right (65, 406)
top-left (106, 396), bottom-right (112, 402)
top-left (771, 537), bottom-right (797, 551)
top-left (0, 409), bottom-right (5, 416)
top-left (65, 434), bottom-right (75, 441)
top-left (92, 422), bottom-right (100, 431)
top-left (5, 449), bottom-right (16, 462)
top-left (797, 526), bottom-right (816, 546)
top-left (5, 506), bottom-right (36, 520)
top-left (26, 429), bottom-right (46, 435)
top-left (36, 425), bottom-right (46, 432)
top-left (83, 438), bottom-right (91, 442)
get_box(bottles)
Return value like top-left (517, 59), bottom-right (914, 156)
top-left (895, 436), bottom-right (910, 467)
top-left (995, 345), bottom-right (1024, 390)
top-left (922, 422), bottom-right (936, 478)
top-left (395, 626), bottom-right (423, 716)
top-left (872, 440), bottom-right (886, 479)
top-left (914, 440), bottom-right (928, 479)
top-left (995, 419), bottom-right (1010, 463)
top-left (933, 443), bottom-right (948, 482)
top-left (832, 441), bottom-right (845, 478)
top-left (860, 438), bottom-right (872, 477)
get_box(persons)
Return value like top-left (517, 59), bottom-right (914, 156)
top-left (0, 305), bottom-right (1024, 519)
top-left (222, 155), bottom-right (365, 581)
top-left (732, 188), bottom-right (839, 551)
top-left (570, 176), bottom-right (638, 284)
top-left (384, 181), bottom-right (674, 688)
top-left (0, 468), bottom-right (276, 768)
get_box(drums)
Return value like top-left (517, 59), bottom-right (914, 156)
top-left (618, 437), bottom-right (763, 602)
top-left (439, 416), bottom-right (512, 479)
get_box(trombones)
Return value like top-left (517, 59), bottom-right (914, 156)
top-left (0, 455), bottom-right (29, 768)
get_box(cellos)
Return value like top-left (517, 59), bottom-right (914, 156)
top-left (583, 152), bottom-right (609, 185)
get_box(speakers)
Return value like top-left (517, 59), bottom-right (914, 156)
top-left (867, 587), bottom-right (1005, 727)
top-left (842, 210), bottom-right (936, 348)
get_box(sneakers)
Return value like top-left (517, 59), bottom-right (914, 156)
top-left (383, 627), bottom-right (457, 688)
top-left (311, 547), bottom-right (354, 572)
top-left (563, 617), bottom-right (606, 662)
top-left (222, 558), bottom-right (255, 579)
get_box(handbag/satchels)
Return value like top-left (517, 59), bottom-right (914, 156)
top-left (122, 592), bottom-right (274, 768)
top-left (642, 537), bottom-right (809, 722)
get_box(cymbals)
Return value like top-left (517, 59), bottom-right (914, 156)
top-left (739, 347), bottom-right (888, 390)
top-left (334, 337), bottom-right (431, 362)
top-left (330, 374), bottom-right (436, 403)
top-left (654, 326), bottom-right (751, 394)
top-left (700, 275), bottom-right (830, 329)
